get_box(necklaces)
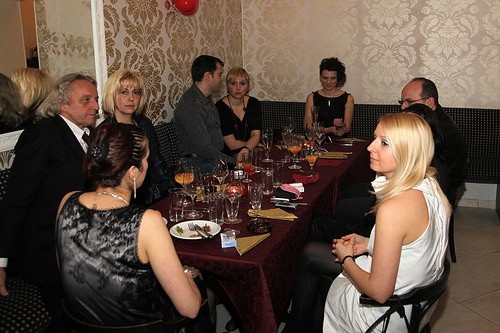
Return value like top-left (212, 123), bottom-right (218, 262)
top-left (226, 95), bottom-right (248, 140)
top-left (97, 191), bottom-right (131, 205)
top-left (323, 91), bottom-right (335, 107)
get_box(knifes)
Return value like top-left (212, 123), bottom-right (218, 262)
top-left (195, 224), bottom-right (214, 238)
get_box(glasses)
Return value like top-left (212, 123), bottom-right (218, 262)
top-left (399, 97), bottom-right (436, 106)
top-left (320, 75), bottom-right (337, 81)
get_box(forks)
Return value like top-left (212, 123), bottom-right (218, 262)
top-left (188, 223), bottom-right (208, 238)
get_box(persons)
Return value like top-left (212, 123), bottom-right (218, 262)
top-left (334, 77), bottom-right (467, 221)
top-left (0, 73), bottom-right (28, 134)
top-left (11, 67), bottom-right (55, 131)
top-left (215, 66), bottom-right (263, 162)
top-left (293, 111), bottom-right (454, 333)
top-left (174, 54), bottom-right (235, 176)
top-left (0, 71), bottom-right (101, 297)
top-left (95, 69), bottom-right (177, 206)
top-left (309, 102), bottom-right (454, 242)
top-left (303, 56), bottom-right (354, 137)
top-left (53, 123), bottom-right (203, 333)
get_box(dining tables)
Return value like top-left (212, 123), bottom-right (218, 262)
top-left (235, 232), bottom-right (271, 256)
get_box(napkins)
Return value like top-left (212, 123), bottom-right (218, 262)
top-left (293, 174), bottom-right (320, 184)
top-left (196, 185), bottom-right (229, 202)
top-left (247, 208), bottom-right (298, 222)
top-left (148, 138), bottom-right (373, 333)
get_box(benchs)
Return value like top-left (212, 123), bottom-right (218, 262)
top-left (0, 101), bottom-right (500, 333)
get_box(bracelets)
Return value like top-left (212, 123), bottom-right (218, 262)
top-left (240, 145), bottom-right (253, 151)
top-left (340, 254), bottom-right (356, 269)
top-left (183, 267), bottom-right (196, 278)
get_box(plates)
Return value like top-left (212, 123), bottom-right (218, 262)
top-left (170, 220), bottom-right (221, 240)
top-left (162, 217), bottom-right (168, 225)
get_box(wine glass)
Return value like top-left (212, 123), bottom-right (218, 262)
top-left (174, 157), bottom-right (194, 207)
top-left (204, 172), bottom-right (213, 202)
top-left (282, 106), bottom-right (326, 177)
top-left (235, 147), bottom-right (261, 182)
top-left (224, 170), bottom-right (243, 224)
top-left (212, 154), bottom-right (229, 196)
top-left (182, 167), bottom-right (203, 219)
top-left (248, 181), bottom-right (264, 221)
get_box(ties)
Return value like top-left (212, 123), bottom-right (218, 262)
top-left (82, 132), bottom-right (90, 145)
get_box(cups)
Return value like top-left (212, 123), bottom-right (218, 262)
top-left (210, 193), bottom-right (239, 225)
top-left (272, 162), bottom-right (282, 186)
top-left (169, 187), bottom-right (184, 222)
top-left (263, 169), bottom-right (274, 194)
top-left (262, 129), bottom-right (272, 152)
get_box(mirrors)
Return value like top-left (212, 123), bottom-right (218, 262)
top-left (0, 0), bottom-right (107, 153)
